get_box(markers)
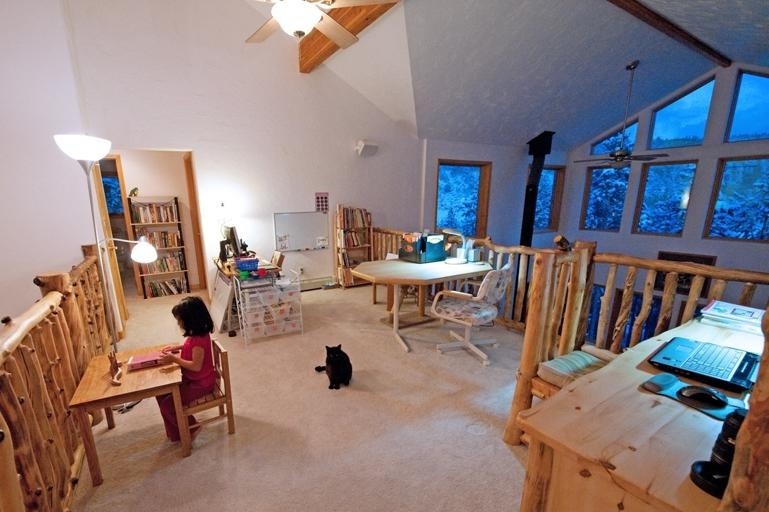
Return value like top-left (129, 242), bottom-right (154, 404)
top-left (305, 248), bottom-right (309, 250)
top-left (320, 247), bottom-right (325, 248)
top-left (297, 249), bottom-right (301, 251)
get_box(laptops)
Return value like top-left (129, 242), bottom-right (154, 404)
top-left (648, 336), bottom-right (761, 391)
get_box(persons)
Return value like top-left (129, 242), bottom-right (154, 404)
top-left (155, 295), bottom-right (214, 446)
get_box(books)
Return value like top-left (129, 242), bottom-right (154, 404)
top-left (125, 352), bottom-right (173, 371)
top-left (335, 205), bottom-right (370, 286)
top-left (700, 299), bottom-right (766, 337)
top-left (130, 203), bottom-right (188, 299)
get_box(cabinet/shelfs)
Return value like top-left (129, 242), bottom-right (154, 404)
top-left (128, 196), bottom-right (190, 299)
top-left (333, 203), bottom-right (374, 288)
top-left (233, 270), bottom-right (305, 348)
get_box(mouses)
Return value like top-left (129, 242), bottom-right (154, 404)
top-left (677, 386), bottom-right (728, 408)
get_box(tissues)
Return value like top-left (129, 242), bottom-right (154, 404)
top-left (456, 239), bottom-right (481, 262)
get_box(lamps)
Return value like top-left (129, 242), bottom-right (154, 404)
top-left (354, 141), bottom-right (378, 157)
top-left (442, 228), bottom-right (468, 264)
top-left (55, 134), bottom-right (159, 352)
top-left (608, 159), bottom-right (631, 169)
top-left (271, 1), bottom-right (320, 39)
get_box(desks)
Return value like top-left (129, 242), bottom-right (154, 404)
top-left (69, 343), bottom-right (190, 488)
top-left (350, 260), bottom-right (494, 353)
top-left (513, 316), bottom-right (765, 512)
top-left (214, 254), bottom-right (282, 330)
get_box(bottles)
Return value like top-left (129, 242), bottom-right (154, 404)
top-left (709, 409), bottom-right (750, 474)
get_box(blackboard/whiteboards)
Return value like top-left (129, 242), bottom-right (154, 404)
top-left (273, 211), bottom-right (329, 252)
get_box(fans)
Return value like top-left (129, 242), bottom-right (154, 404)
top-left (574, 61), bottom-right (669, 166)
top-left (245, 0), bottom-right (401, 49)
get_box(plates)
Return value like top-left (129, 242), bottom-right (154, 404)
top-left (690, 458), bottom-right (729, 502)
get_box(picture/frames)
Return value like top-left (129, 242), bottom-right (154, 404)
top-left (653, 251), bottom-right (717, 299)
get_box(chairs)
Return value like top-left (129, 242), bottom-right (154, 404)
top-left (182, 338), bottom-right (236, 450)
top-left (502, 248), bottom-right (607, 449)
top-left (270, 251), bottom-right (285, 267)
top-left (429, 258), bottom-right (513, 367)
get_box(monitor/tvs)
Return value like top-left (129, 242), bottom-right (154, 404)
top-left (219, 226), bottom-right (241, 262)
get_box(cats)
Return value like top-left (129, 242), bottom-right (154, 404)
top-left (314, 344), bottom-right (352, 391)
